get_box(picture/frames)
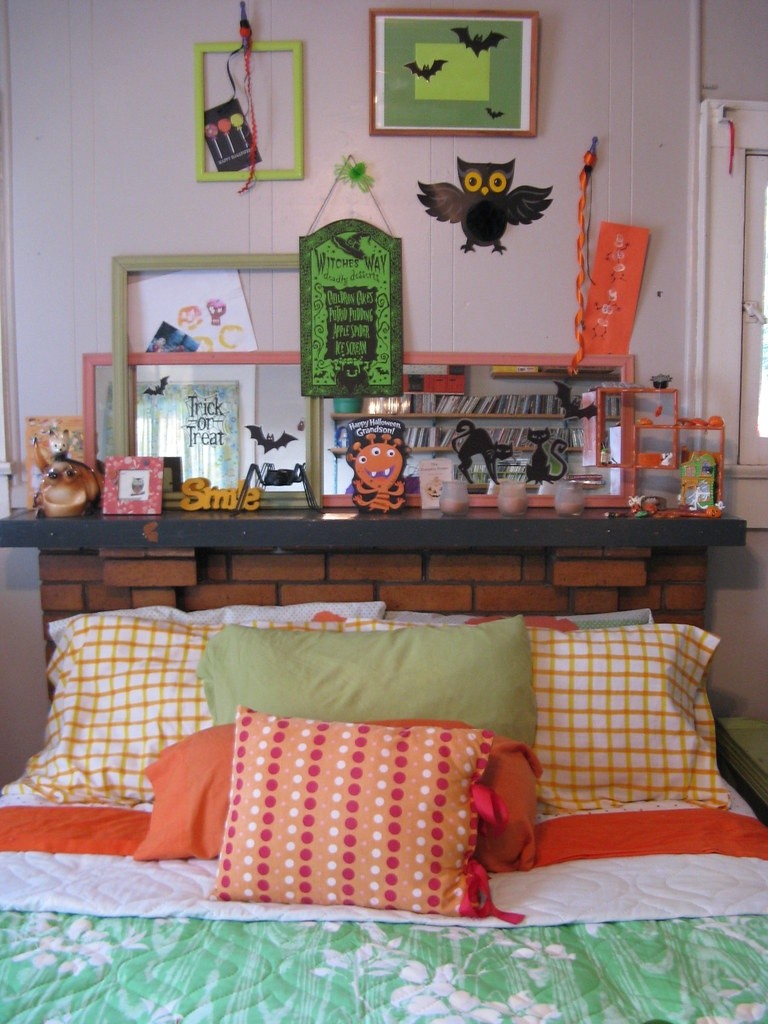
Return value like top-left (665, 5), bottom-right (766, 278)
top-left (192, 40), bottom-right (306, 184)
top-left (161, 456), bottom-right (184, 494)
top-left (25, 416), bottom-right (85, 511)
top-left (101, 455), bottom-right (163, 516)
top-left (367, 5), bottom-right (539, 138)
top-left (110, 250), bottom-right (325, 513)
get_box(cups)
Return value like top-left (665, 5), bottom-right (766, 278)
top-left (439, 480), bottom-right (469, 515)
top-left (554, 480), bottom-right (586, 516)
top-left (498, 480), bottom-right (528, 515)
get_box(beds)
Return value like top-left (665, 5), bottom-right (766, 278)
top-left (0, 775), bottom-right (767, 1023)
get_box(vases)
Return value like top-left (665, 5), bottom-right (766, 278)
top-left (334, 396), bottom-right (363, 412)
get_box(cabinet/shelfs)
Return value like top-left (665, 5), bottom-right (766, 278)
top-left (329, 411), bottom-right (622, 497)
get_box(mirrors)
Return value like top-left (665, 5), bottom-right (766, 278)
top-left (81, 350), bottom-right (636, 508)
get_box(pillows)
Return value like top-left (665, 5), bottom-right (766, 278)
top-left (202, 704), bottom-right (525, 926)
top-left (197, 613), bottom-right (538, 748)
top-left (2, 615), bottom-right (341, 805)
top-left (344, 618), bottom-right (732, 815)
top-left (48, 600), bottom-right (387, 642)
top-left (384, 608), bottom-right (654, 629)
top-left (131, 716), bottom-right (541, 874)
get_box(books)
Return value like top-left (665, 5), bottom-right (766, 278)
top-left (359, 392), bottom-right (627, 491)
